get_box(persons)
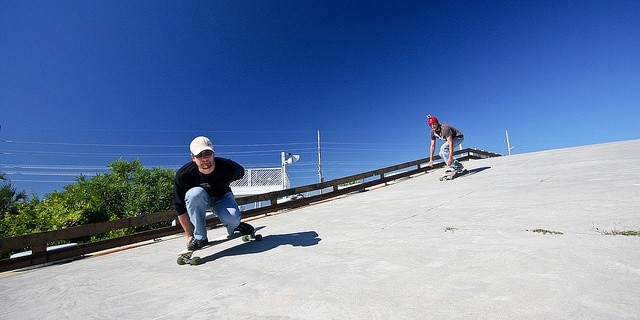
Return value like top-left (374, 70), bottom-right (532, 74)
top-left (172, 135), bottom-right (255, 250)
top-left (426, 116), bottom-right (464, 175)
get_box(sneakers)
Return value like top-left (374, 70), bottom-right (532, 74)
top-left (234, 222), bottom-right (254, 234)
top-left (445, 165), bottom-right (463, 174)
top-left (188, 238), bottom-right (208, 250)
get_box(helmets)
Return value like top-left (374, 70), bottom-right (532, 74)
top-left (428, 116), bottom-right (438, 126)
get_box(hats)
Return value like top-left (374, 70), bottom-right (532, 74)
top-left (190, 136), bottom-right (215, 155)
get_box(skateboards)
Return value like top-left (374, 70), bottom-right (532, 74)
top-left (439, 170), bottom-right (469, 181)
top-left (177, 226), bottom-right (266, 265)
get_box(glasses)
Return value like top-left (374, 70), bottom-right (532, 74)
top-left (195, 153), bottom-right (212, 159)
